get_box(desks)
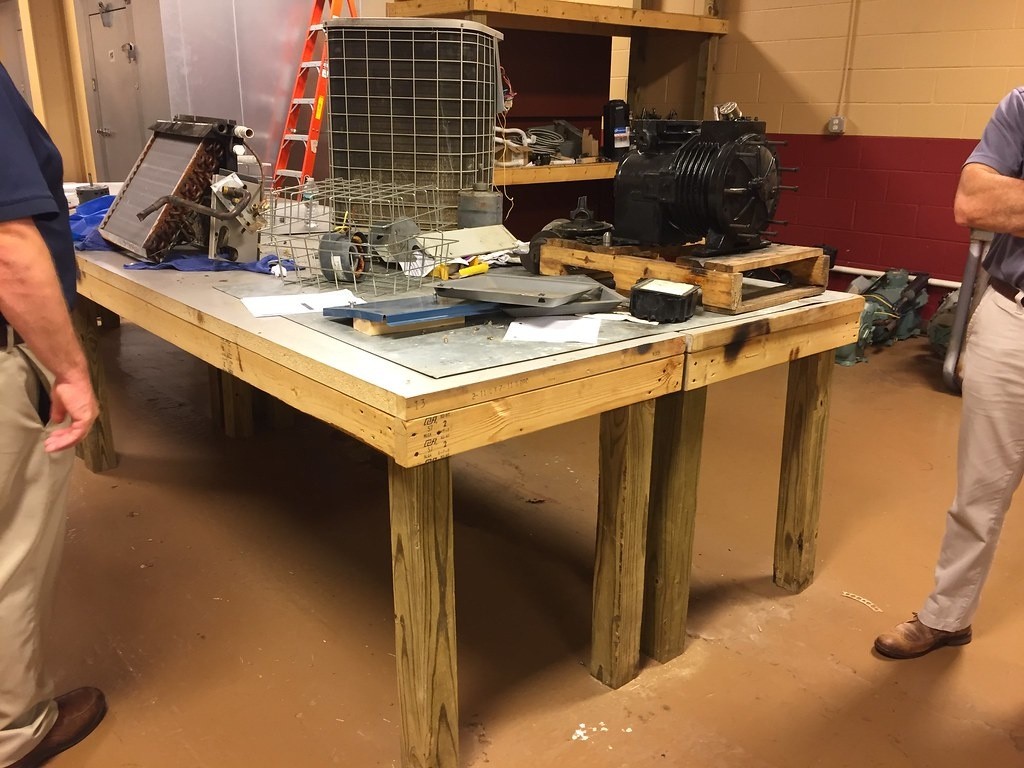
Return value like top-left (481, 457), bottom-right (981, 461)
top-left (67, 243), bottom-right (688, 768)
top-left (624, 277), bottom-right (865, 664)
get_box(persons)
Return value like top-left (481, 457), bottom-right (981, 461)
top-left (0, 62), bottom-right (112, 766)
top-left (870, 89), bottom-right (1023, 660)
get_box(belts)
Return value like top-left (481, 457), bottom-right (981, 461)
top-left (0, 324), bottom-right (24, 345)
top-left (991, 278), bottom-right (1024, 306)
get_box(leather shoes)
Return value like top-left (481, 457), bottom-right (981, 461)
top-left (2, 686), bottom-right (106, 768)
top-left (876, 612), bottom-right (972, 658)
